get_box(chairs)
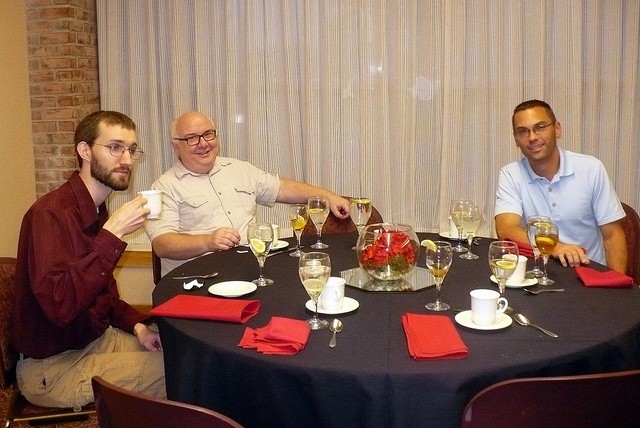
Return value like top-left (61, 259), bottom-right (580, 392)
top-left (90, 375), bottom-right (240, 427)
top-left (462, 370), bottom-right (639, 426)
top-left (301, 196), bottom-right (384, 239)
top-left (614, 199), bottom-right (639, 287)
top-left (148, 241), bottom-right (164, 288)
top-left (1, 254), bottom-right (124, 426)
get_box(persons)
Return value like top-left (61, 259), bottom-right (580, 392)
top-left (12, 110), bottom-right (168, 405)
top-left (493, 99), bottom-right (628, 277)
top-left (141, 110), bottom-right (351, 279)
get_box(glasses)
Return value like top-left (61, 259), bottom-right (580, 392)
top-left (87, 142), bottom-right (144, 160)
top-left (174, 129), bottom-right (216, 146)
top-left (514, 122), bottom-right (553, 137)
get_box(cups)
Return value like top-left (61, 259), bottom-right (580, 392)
top-left (137, 189), bottom-right (163, 220)
top-left (501, 252), bottom-right (528, 285)
top-left (254, 224), bottom-right (279, 247)
top-left (469, 289), bottom-right (508, 326)
top-left (322, 277), bottom-right (346, 310)
top-left (448, 215), bottom-right (459, 237)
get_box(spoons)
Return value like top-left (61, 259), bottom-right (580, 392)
top-left (513, 312), bottom-right (559, 338)
top-left (171, 271), bottom-right (218, 281)
top-left (328, 318), bottom-right (344, 349)
top-left (523, 286), bottom-right (566, 295)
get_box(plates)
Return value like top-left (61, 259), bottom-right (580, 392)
top-left (489, 273), bottom-right (539, 289)
top-left (454, 309), bottom-right (514, 332)
top-left (262, 239), bottom-right (290, 251)
top-left (304, 296), bottom-right (360, 315)
top-left (438, 230), bottom-right (474, 242)
top-left (207, 281), bottom-right (258, 298)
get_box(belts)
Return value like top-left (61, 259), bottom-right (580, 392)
top-left (16, 353), bottom-right (32, 360)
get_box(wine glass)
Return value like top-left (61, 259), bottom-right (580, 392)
top-left (488, 240), bottom-right (519, 313)
top-left (309, 197), bottom-right (330, 249)
top-left (450, 200), bottom-right (472, 253)
top-left (288, 205), bottom-right (309, 258)
top-left (425, 241), bottom-right (453, 312)
top-left (534, 223), bottom-right (559, 286)
top-left (246, 222), bottom-right (274, 288)
top-left (526, 216), bottom-right (550, 278)
top-left (348, 198), bottom-right (373, 251)
top-left (298, 252), bottom-right (331, 331)
top-left (459, 204), bottom-right (480, 261)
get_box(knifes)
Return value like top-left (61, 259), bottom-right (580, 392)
top-left (265, 245), bottom-right (305, 258)
